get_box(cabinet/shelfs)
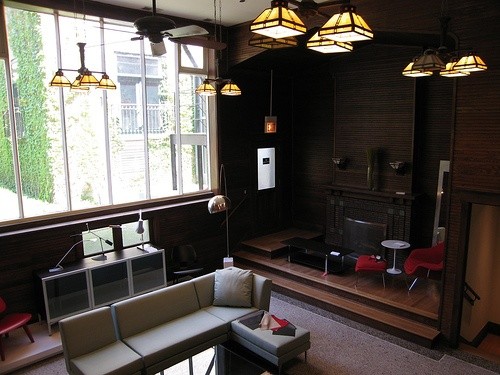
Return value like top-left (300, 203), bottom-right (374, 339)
top-left (34, 243), bottom-right (168, 336)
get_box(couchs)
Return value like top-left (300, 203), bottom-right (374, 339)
top-left (58, 265), bottom-right (311, 375)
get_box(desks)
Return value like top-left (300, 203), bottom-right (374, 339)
top-left (381, 239), bottom-right (412, 274)
top-left (280, 236), bottom-right (354, 276)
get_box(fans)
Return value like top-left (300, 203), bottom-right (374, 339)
top-left (95, 0), bottom-right (210, 58)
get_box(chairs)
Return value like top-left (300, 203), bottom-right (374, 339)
top-left (403, 240), bottom-right (445, 291)
top-left (0, 294), bottom-right (35, 362)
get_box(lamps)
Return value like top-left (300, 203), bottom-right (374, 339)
top-left (401, 46), bottom-right (489, 79)
top-left (248, 0), bottom-right (373, 55)
top-left (208, 194), bottom-right (232, 216)
top-left (195, 76), bottom-right (243, 96)
top-left (48, 40), bottom-right (117, 92)
